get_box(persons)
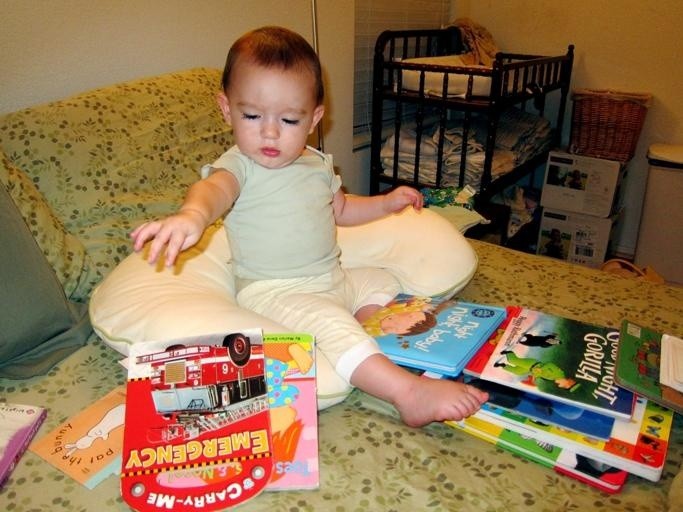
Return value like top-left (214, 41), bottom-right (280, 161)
top-left (546, 164), bottom-right (567, 187)
top-left (125, 23), bottom-right (490, 429)
top-left (541, 228), bottom-right (566, 261)
top-left (567, 168), bottom-right (585, 192)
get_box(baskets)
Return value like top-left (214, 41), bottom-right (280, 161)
top-left (567, 89), bottom-right (652, 163)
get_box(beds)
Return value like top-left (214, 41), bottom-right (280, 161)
top-left (0, 237), bottom-right (683, 511)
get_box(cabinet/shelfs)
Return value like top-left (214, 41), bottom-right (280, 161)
top-left (369, 27), bottom-right (575, 250)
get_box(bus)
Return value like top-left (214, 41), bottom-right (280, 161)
top-left (133, 333), bottom-right (265, 429)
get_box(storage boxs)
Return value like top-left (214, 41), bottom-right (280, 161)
top-left (536, 147), bottom-right (629, 271)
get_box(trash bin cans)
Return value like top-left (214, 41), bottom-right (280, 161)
top-left (633, 142), bottom-right (683, 285)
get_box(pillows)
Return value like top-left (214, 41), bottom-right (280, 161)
top-left (0, 178), bottom-right (75, 369)
top-left (0, 148), bottom-right (104, 304)
top-left (0, 69), bottom-right (236, 279)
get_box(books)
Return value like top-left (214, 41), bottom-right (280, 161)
top-left (261, 333), bottom-right (320, 489)
top-left (362, 294), bottom-right (682, 495)
top-left (1, 400), bottom-right (51, 489)
